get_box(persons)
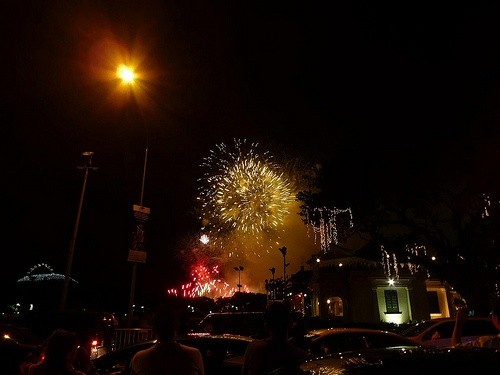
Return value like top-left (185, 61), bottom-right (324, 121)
top-left (22, 328), bottom-right (102, 375)
top-left (129, 312), bottom-right (206, 375)
top-left (240, 309), bottom-right (309, 375)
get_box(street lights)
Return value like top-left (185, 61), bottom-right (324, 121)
top-left (233, 265), bottom-right (243, 293)
top-left (278, 246), bottom-right (290, 300)
top-left (54, 150), bottom-right (97, 333)
top-left (120, 66), bottom-right (150, 328)
top-left (268, 267), bottom-right (275, 301)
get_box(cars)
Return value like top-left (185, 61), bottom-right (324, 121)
top-left (0, 300), bottom-right (500, 375)
